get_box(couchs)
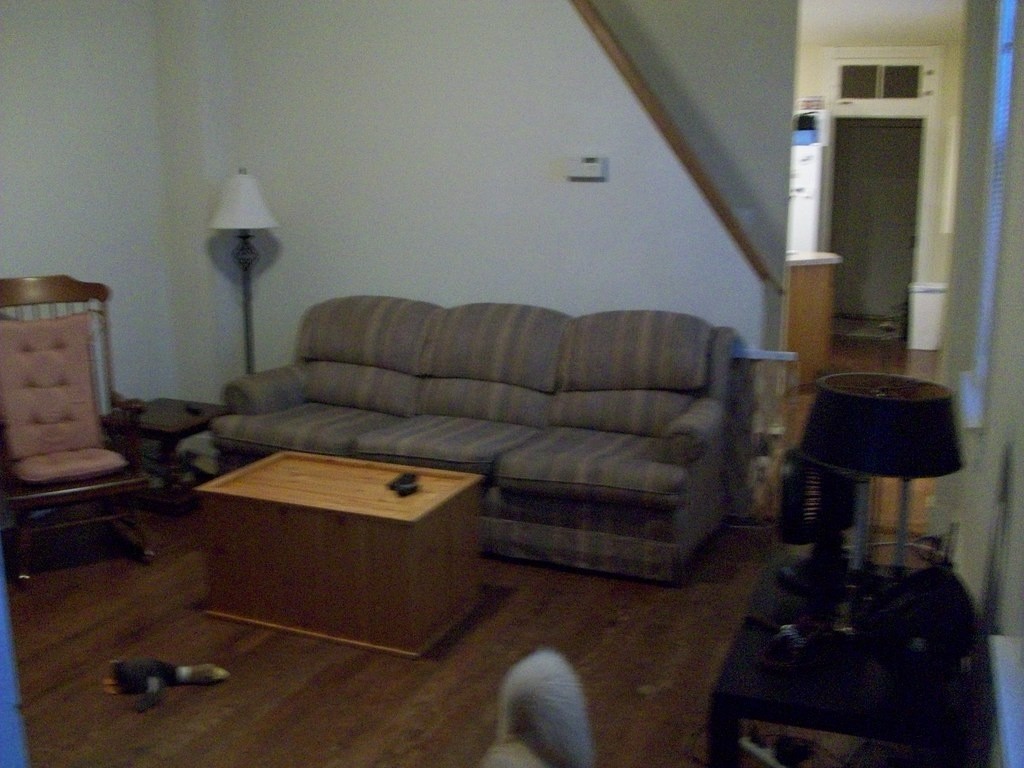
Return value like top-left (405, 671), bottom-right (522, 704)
top-left (212, 294), bottom-right (746, 582)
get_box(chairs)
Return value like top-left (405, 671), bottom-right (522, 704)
top-left (0, 273), bottom-right (155, 583)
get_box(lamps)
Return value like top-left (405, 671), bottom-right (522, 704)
top-left (798, 376), bottom-right (960, 643)
top-left (209, 165), bottom-right (278, 376)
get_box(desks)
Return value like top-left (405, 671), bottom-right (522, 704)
top-left (111, 397), bottom-right (226, 509)
top-left (707, 548), bottom-right (995, 767)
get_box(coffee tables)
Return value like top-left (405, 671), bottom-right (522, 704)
top-left (192, 451), bottom-right (486, 660)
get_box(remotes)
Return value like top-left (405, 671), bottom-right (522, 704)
top-left (385, 471), bottom-right (419, 496)
